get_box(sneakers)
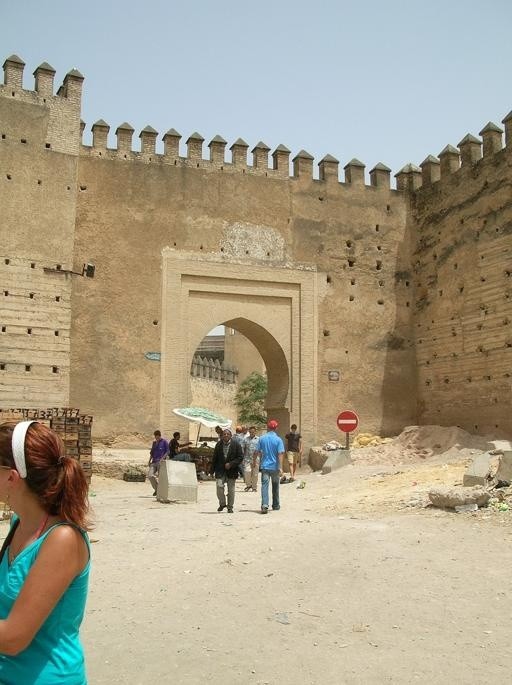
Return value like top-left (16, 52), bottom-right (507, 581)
top-left (245, 485), bottom-right (257, 492)
top-left (289, 478), bottom-right (293, 482)
top-left (153, 490), bottom-right (157, 496)
top-left (218, 505), bottom-right (226, 511)
top-left (261, 507), bottom-right (267, 514)
top-left (228, 509), bottom-right (233, 513)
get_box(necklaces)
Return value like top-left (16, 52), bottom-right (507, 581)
top-left (10, 523), bottom-right (41, 562)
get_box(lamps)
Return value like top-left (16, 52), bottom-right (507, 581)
top-left (71, 263), bottom-right (95, 278)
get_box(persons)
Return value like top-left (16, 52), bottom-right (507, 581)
top-left (241, 426), bottom-right (252, 439)
top-left (208, 428), bottom-right (245, 514)
top-left (241, 426), bottom-right (261, 492)
top-left (253, 419), bottom-right (285, 516)
top-left (285, 424), bottom-right (303, 484)
top-left (215, 426), bottom-right (224, 439)
top-left (231, 426), bottom-right (247, 480)
top-left (169, 432), bottom-right (194, 463)
top-left (150, 431), bottom-right (171, 497)
top-left (1, 420), bottom-right (92, 685)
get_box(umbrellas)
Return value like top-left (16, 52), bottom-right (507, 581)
top-left (173, 406), bottom-right (233, 448)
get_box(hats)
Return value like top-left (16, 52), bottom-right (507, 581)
top-left (268, 420), bottom-right (278, 429)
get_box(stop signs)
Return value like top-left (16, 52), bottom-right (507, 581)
top-left (336, 410), bottom-right (359, 432)
